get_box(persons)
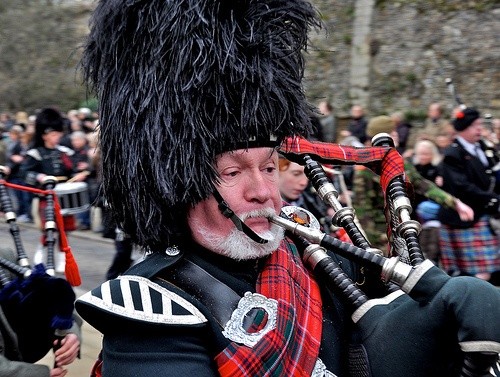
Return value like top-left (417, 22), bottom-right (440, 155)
top-left (0, 298), bottom-right (84, 376)
top-left (16, 109), bottom-right (95, 274)
top-left (434, 103), bottom-right (500, 282)
top-left (1, 96), bottom-right (500, 245)
top-left (73, 96), bottom-right (500, 377)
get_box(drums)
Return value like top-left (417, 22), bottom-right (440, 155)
top-left (46, 181), bottom-right (90, 230)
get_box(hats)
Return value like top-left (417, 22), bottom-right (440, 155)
top-left (82, 0), bottom-right (328, 246)
top-left (33, 107), bottom-right (66, 146)
top-left (455, 108), bottom-right (480, 132)
top-left (367, 116), bottom-right (397, 137)
